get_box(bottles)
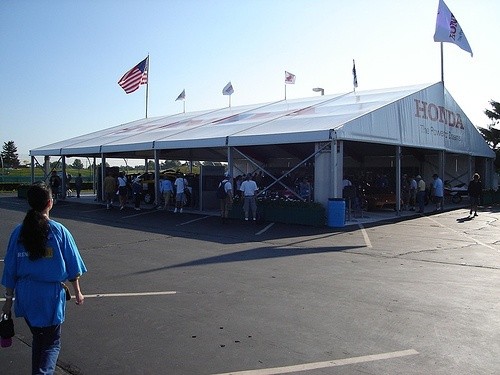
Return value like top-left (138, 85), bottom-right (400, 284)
top-left (0, 313), bottom-right (12, 348)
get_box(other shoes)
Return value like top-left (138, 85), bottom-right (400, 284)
top-left (120, 206), bottom-right (123, 210)
top-left (245, 217), bottom-right (249, 220)
top-left (253, 218), bottom-right (256, 220)
top-left (174, 208), bottom-right (177, 212)
top-left (180, 208), bottom-right (183, 213)
top-left (134, 208), bottom-right (141, 210)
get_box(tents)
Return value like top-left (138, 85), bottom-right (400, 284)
top-left (29, 84), bottom-right (500, 217)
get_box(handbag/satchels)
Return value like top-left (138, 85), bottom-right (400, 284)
top-left (184, 188), bottom-right (191, 198)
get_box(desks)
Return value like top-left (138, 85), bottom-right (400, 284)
top-left (375, 196), bottom-right (404, 209)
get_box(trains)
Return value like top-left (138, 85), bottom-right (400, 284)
top-left (0, 174), bottom-right (97, 192)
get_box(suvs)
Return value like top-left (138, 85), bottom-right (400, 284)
top-left (126, 169), bottom-right (194, 209)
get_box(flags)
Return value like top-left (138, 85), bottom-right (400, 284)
top-left (175, 89), bottom-right (185, 101)
top-left (285, 71), bottom-right (295, 84)
top-left (352, 61), bottom-right (358, 87)
top-left (118, 57), bottom-right (148, 94)
top-left (433, 0), bottom-right (473, 58)
top-left (222, 82), bottom-right (234, 95)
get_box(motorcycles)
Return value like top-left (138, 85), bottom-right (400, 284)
top-left (429, 180), bottom-right (467, 204)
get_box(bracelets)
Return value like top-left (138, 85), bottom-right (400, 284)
top-left (232, 197), bottom-right (233, 199)
top-left (5, 293), bottom-right (13, 300)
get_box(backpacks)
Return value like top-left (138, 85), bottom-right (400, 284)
top-left (216, 181), bottom-right (230, 199)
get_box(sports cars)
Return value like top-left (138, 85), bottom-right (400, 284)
top-left (366, 184), bottom-right (405, 212)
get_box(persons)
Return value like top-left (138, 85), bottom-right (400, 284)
top-left (467, 174), bottom-right (482, 216)
top-left (234, 171), bottom-right (314, 201)
top-left (408, 175), bottom-right (425, 213)
top-left (240, 174), bottom-right (258, 221)
top-left (350, 173), bottom-right (412, 211)
top-left (430, 172), bottom-right (443, 213)
top-left (103, 170), bottom-right (143, 212)
top-left (156, 172), bottom-right (188, 213)
top-left (49, 171), bottom-right (83, 200)
top-left (218, 175), bottom-right (235, 217)
top-left (0, 180), bottom-right (88, 375)
top-left (342, 174), bottom-right (353, 221)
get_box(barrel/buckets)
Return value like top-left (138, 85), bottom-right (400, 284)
top-left (327, 198), bottom-right (346, 227)
